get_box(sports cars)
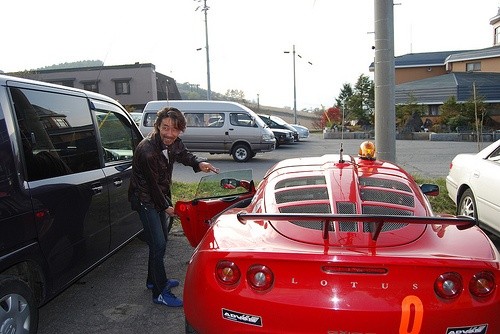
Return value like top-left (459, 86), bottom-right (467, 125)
top-left (174, 141), bottom-right (500, 334)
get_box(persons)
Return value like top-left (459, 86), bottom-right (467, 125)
top-left (129, 107), bottom-right (220, 307)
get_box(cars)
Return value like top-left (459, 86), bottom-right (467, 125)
top-left (291, 124), bottom-right (309, 138)
top-left (446, 139), bottom-right (500, 237)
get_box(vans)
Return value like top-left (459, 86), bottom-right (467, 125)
top-left (206, 115), bottom-right (297, 144)
top-left (138, 100), bottom-right (277, 162)
top-left (0, 74), bottom-right (172, 334)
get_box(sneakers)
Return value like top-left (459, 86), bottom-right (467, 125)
top-left (152, 286), bottom-right (183, 307)
top-left (147, 279), bottom-right (179, 289)
